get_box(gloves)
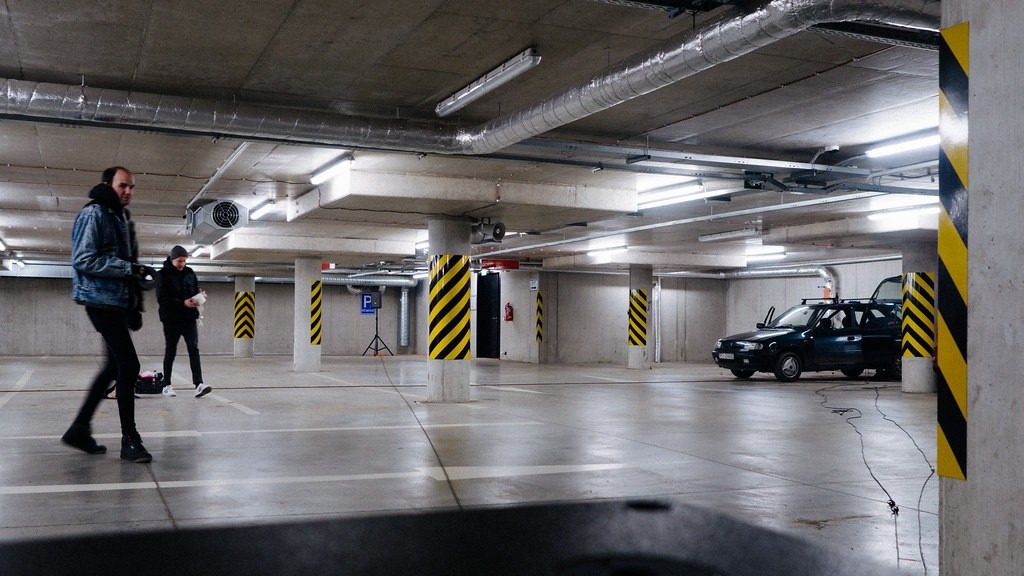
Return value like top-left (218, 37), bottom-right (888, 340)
top-left (124, 310), bottom-right (142, 331)
top-left (131, 264), bottom-right (158, 290)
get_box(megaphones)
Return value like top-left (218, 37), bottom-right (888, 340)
top-left (478, 221), bottom-right (505, 241)
top-left (470, 225), bottom-right (485, 244)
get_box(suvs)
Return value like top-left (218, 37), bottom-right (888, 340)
top-left (712, 274), bottom-right (902, 383)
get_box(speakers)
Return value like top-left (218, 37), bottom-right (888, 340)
top-left (371, 291), bottom-right (382, 309)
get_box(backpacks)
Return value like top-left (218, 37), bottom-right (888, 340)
top-left (135, 370), bottom-right (165, 394)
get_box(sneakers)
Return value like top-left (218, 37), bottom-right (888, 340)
top-left (162, 385), bottom-right (176, 397)
top-left (120, 436), bottom-right (152, 463)
top-left (194, 383), bottom-right (212, 398)
top-left (61, 427), bottom-right (108, 454)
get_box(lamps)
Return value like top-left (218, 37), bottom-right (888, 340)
top-left (435, 48), bottom-right (542, 118)
top-left (310, 155), bottom-right (355, 186)
top-left (250, 200), bottom-right (276, 221)
top-left (698, 228), bottom-right (759, 243)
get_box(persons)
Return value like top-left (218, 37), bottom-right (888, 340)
top-left (60, 166), bottom-right (157, 464)
top-left (155, 245), bottom-right (212, 398)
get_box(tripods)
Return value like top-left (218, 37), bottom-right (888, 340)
top-left (363, 308), bottom-right (394, 357)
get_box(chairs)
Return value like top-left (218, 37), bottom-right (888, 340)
top-left (816, 317), bottom-right (832, 330)
top-left (841, 316), bottom-right (852, 329)
